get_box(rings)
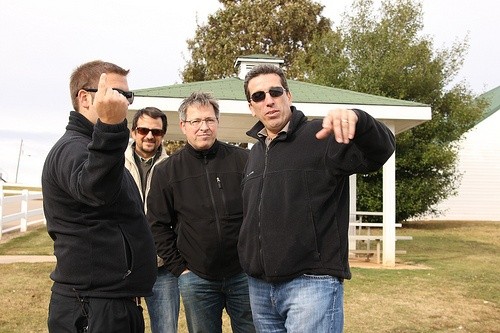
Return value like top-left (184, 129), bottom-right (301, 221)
top-left (342, 119), bottom-right (348, 123)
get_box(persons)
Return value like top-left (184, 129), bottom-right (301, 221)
top-left (41, 60), bottom-right (158, 333)
top-left (143, 92), bottom-right (256, 333)
top-left (125, 107), bottom-right (180, 333)
top-left (238, 66), bottom-right (395, 333)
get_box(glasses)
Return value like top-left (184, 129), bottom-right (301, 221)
top-left (184, 118), bottom-right (215, 126)
top-left (248, 87), bottom-right (286, 103)
top-left (75, 88), bottom-right (135, 106)
top-left (136, 127), bottom-right (164, 136)
